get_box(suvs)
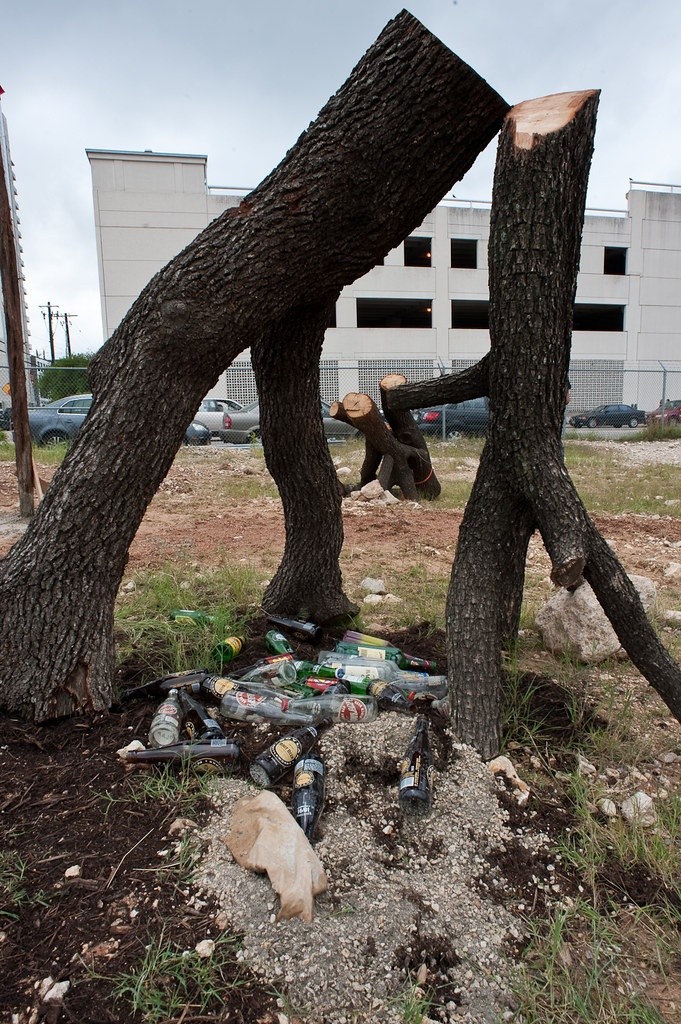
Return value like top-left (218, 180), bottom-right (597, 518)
top-left (645, 400), bottom-right (681, 433)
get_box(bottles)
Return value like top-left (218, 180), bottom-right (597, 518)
top-left (127, 609), bottom-right (449, 843)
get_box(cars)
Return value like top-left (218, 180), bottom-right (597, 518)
top-left (12, 392), bottom-right (212, 450)
top-left (218, 398), bottom-right (366, 450)
top-left (412, 398), bottom-right (489, 443)
top-left (568, 404), bottom-right (642, 428)
top-left (193, 398), bottom-right (245, 436)
